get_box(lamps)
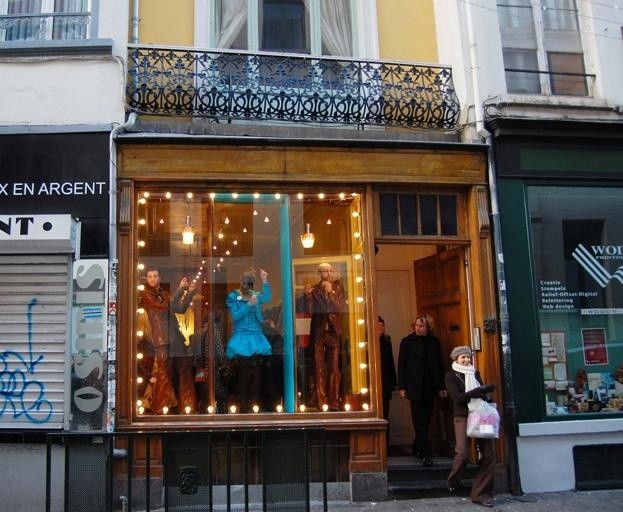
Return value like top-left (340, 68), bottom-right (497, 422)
top-left (180, 214), bottom-right (197, 245)
top-left (301, 222), bottom-right (315, 249)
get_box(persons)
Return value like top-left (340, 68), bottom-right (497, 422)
top-left (189, 308), bottom-right (230, 408)
top-left (364, 315), bottom-right (397, 456)
top-left (140, 264), bottom-right (177, 416)
top-left (174, 274), bottom-right (200, 415)
top-left (312, 263), bottom-right (345, 410)
top-left (397, 313), bottom-right (441, 466)
top-left (223, 265), bottom-right (271, 411)
top-left (444, 345), bottom-right (496, 507)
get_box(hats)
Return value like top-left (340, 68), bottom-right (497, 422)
top-left (450, 346), bottom-right (472, 360)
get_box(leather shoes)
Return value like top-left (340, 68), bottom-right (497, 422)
top-left (472, 500), bottom-right (493, 507)
top-left (411, 451), bottom-right (420, 457)
top-left (448, 487), bottom-right (456, 496)
top-left (423, 458), bottom-right (433, 464)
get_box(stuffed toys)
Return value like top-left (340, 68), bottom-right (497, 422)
top-left (566, 364), bottom-right (623, 413)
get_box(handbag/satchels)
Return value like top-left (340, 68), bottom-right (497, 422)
top-left (193, 367), bottom-right (209, 383)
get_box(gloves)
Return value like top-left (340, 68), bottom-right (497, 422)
top-left (480, 383), bottom-right (495, 393)
top-left (482, 393), bottom-right (493, 403)
